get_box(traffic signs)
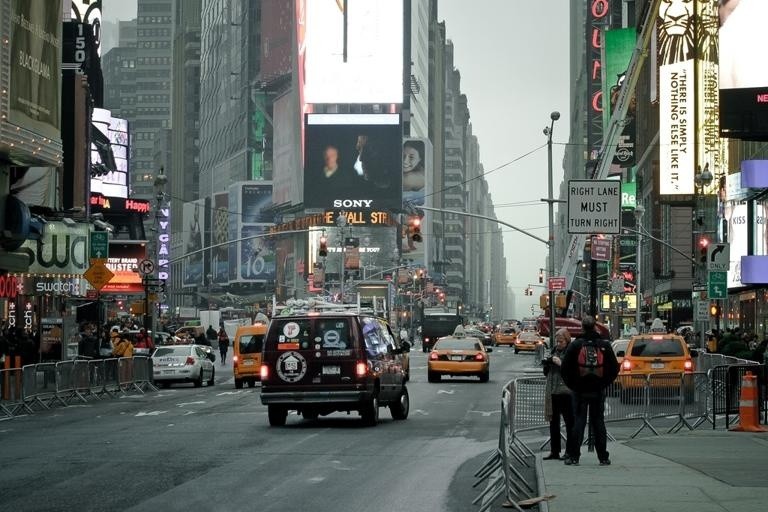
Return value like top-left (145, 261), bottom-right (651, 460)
top-left (86, 227), bottom-right (111, 260)
top-left (705, 243), bottom-right (733, 271)
top-left (707, 271), bottom-right (731, 302)
top-left (136, 259), bottom-right (154, 277)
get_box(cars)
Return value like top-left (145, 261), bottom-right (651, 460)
top-left (151, 345), bottom-right (214, 388)
top-left (437, 329), bottom-right (492, 352)
top-left (514, 332), bottom-right (544, 353)
top-left (427, 325), bottom-right (489, 383)
top-left (607, 340), bottom-right (630, 399)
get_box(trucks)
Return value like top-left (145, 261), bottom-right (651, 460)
top-left (420, 315), bottom-right (464, 352)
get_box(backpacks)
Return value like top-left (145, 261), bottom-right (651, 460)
top-left (577, 336), bottom-right (606, 385)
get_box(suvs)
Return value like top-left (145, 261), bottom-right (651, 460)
top-left (491, 327), bottom-right (516, 347)
top-left (619, 334), bottom-right (696, 405)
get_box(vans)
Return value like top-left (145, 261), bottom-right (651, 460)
top-left (232, 325), bottom-right (269, 389)
top-left (260, 314), bottom-right (409, 427)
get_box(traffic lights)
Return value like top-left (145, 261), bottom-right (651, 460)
top-left (529, 289), bottom-right (532, 297)
top-left (411, 209), bottom-right (424, 244)
top-left (412, 217), bottom-right (422, 242)
top-left (539, 274), bottom-right (544, 284)
top-left (698, 237), bottom-right (711, 270)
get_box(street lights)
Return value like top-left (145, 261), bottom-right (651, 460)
top-left (542, 110), bottom-right (562, 349)
top-left (540, 109), bottom-right (559, 350)
top-left (147, 163), bottom-right (171, 288)
top-left (537, 266), bottom-right (566, 281)
top-left (522, 282), bottom-right (549, 295)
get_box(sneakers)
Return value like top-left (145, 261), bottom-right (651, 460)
top-left (571, 458), bottom-right (579, 465)
top-left (601, 458), bottom-right (611, 465)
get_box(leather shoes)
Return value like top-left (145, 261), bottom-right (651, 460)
top-left (561, 453), bottom-right (568, 460)
top-left (543, 453), bottom-right (559, 460)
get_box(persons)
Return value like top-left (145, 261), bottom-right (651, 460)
top-left (541, 328), bottom-right (577, 460)
top-left (355, 126), bottom-right (402, 196)
top-left (218, 331), bottom-right (229, 364)
top-left (399, 322), bottom-right (425, 345)
top-left (679, 327), bottom-right (768, 365)
top-left (111, 329), bottom-right (121, 380)
top-left (561, 316), bottom-right (620, 466)
top-left (135, 327), bottom-right (155, 350)
top-left (112, 334), bottom-right (133, 382)
top-left (402, 140), bottom-right (426, 206)
top-left (187, 203), bottom-right (202, 264)
